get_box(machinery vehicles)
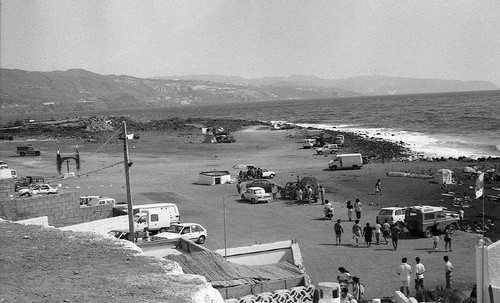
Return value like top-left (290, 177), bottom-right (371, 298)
top-left (334, 134), bottom-right (345, 146)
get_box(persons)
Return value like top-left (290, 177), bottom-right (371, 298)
top-left (347, 201), bottom-right (353, 221)
top-left (382, 219), bottom-right (391, 244)
top-left (271, 183), bottom-right (278, 202)
top-left (337, 267), bottom-right (351, 293)
top-left (444, 225), bottom-right (452, 251)
top-left (443, 255), bottom-right (453, 288)
top-left (352, 220), bottom-right (362, 246)
top-left (375, 179), bottom-right (382, 195)
top-left (237, 167), bottom-right (262, 193)
top-left (397, 257), bottom-right (412, 298)
top-left (432, 232), bottom-right (439, 250)
top-left (348, 276), bottom-right (365, 300)
top-left (414, 256), bottom-right (425, 291)
top-left (362, 223), bottom-right (374, 247)
top-left (391, 222), bottom-right (401, 251)
top-left (374, 220), bottom-right (382, 245)
top-left (334, 219), bottom-right (344, 246)
top-left (354, 199), bottom-right (363, 219)
top-left (324, 199), bottom-right (332, 209)
top-left (296, 184), bottom-right (325, 205)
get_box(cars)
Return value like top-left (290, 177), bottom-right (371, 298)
top-left (79, 196), bottom-right (115, 209)
top-left (240, 187), bottom-right (271, 203)
top-left (17, 184), bottom-right (58, 197)
top-left (246, 178), bottom-right (274, 188)
top-left (0, 161), bottom-right (17, 179)
top-left (254, 169), bottom-right (275, 178)
top-left (152, 223), bottom-right (208, 244)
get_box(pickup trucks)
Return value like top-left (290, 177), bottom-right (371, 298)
top-left (316, 145), bottom-right (339, 155)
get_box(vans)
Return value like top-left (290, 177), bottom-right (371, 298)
top-left (376, 207), bottom-right (407, 225)
top-left (303, 139), bottom-right (316, 149)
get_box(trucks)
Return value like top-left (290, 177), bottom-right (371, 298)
top-left (328, 153), bottom-right (363, 171)
top-left (269, 121), bottom-right (295, 130)
top-left (305, 130), bottom-right (333, 142)
top-left (108, 203), bottom-right (180, 238)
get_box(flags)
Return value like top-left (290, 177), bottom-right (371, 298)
top-left (473, 172), bottom-right (483, 199)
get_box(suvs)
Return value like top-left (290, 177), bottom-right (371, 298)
top-left (16, 175), bottom-right (44, 192)
top-left (280, 181), bottom-right (307, 199)
top-left (16, 145), bottom-right (40, 156)
top-left (405, 205), bottom-right (461, 238)
